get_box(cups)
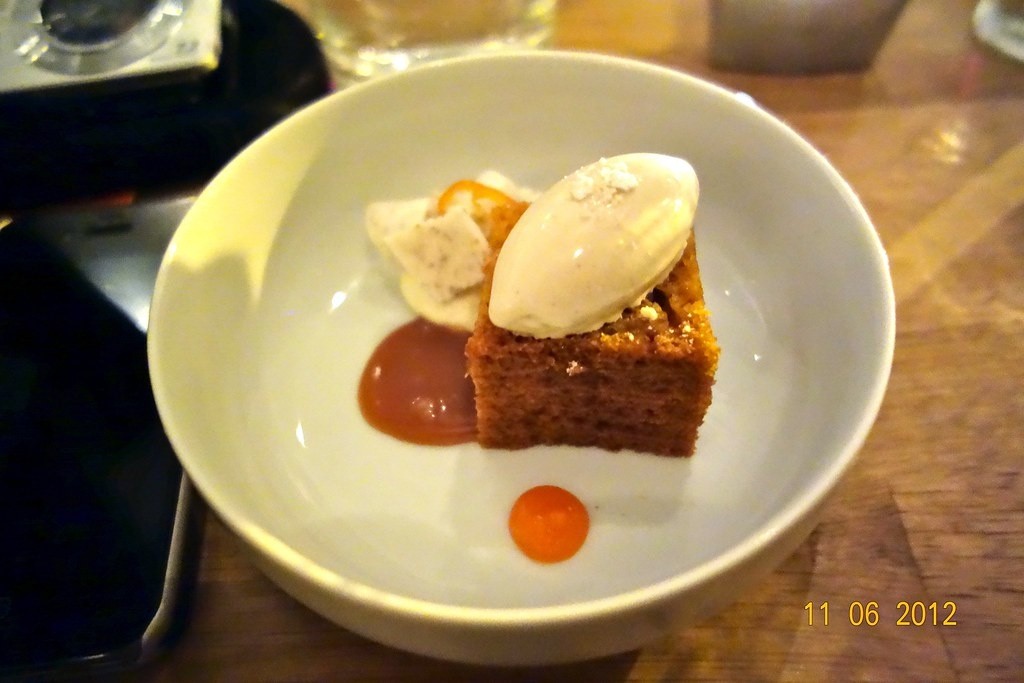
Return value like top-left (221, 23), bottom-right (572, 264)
top-left (306, 0), bottom-right (557, 82)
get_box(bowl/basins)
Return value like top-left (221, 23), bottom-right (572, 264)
top-left (148, 49), bottom-right (898, 671)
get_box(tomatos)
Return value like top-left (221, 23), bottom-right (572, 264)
top-left (509, 485), bottom-right (596, 566)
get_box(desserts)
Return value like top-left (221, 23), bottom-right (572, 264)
top-left (364, 170), bottom-right (539, 334)
top-left (460, 151), bottom-right (722, 460)
